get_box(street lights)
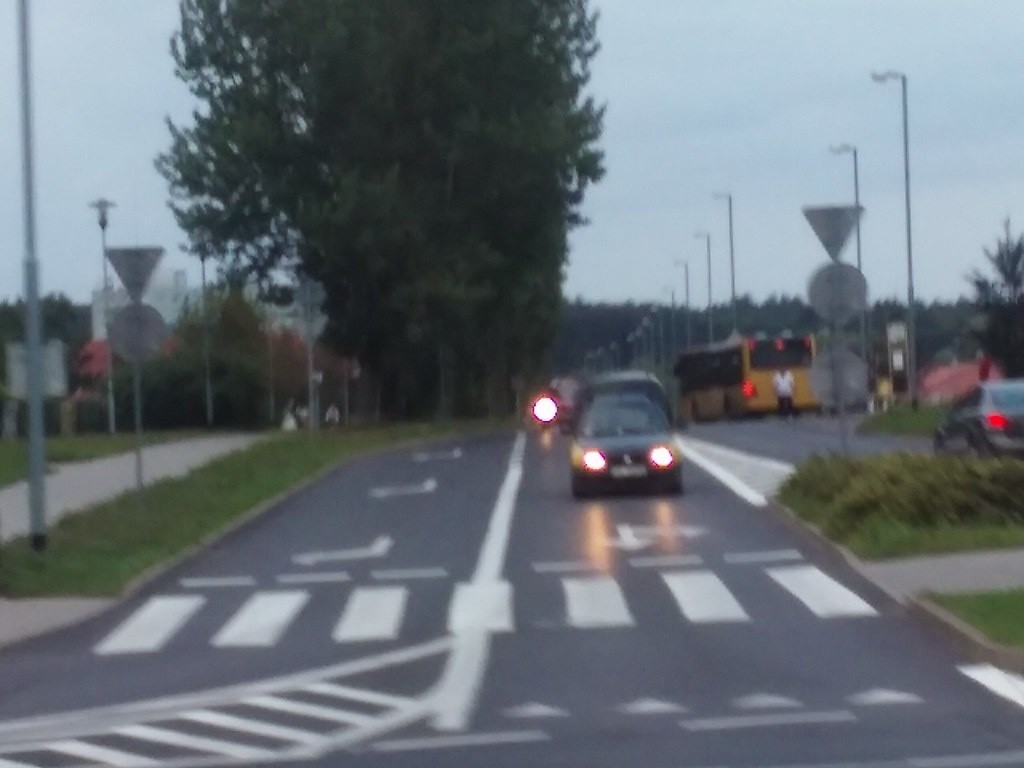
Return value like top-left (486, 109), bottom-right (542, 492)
top-left (873, 70), bottom-right (918, 406)
top-left (828, 143), bottom-right (866, 360)
top-left (583, 192), bottom-right (738, 382)
top-left (87, 198), bottom-right (119, 433)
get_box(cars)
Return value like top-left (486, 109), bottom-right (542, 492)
top-left (933, 382), bottom-right (1024, 459)
top-left (529, 373), bottom-right (676, 434)
top-left (569, 395), bottom-right (681, 497)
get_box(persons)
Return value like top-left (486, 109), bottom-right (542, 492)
top-left (978, 352), bottom-right (993, 379)
top-left (326, 402), bottom-right (339, 422)
top-left (771, 366), bottom-right (799, 416)
top-left (282, 396), bottom-right (309, 430)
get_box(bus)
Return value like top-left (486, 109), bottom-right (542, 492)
top-left (671, 336), bottom-right (816, 425)
